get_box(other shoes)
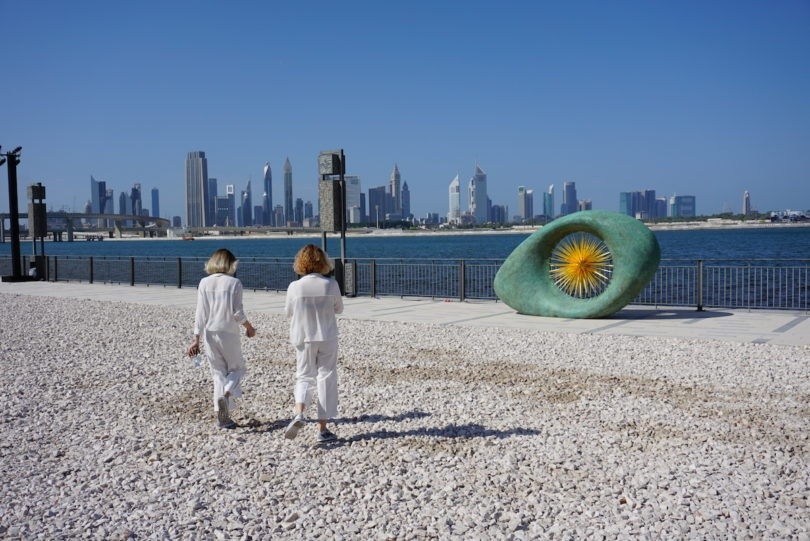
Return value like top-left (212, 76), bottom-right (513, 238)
top-left (216, 417), bottom-right (235, 429)
top-left (217, 396), bottom-right (230, 423)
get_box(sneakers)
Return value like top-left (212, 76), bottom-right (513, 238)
top-left (317, 429), bottom-right (338, 442)
top-left (285, 414), bottom-right (305, 440)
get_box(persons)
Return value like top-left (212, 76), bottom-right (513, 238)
top-left (185, 248), bottom-right (259, 426)
top-left (281, 245), bottom-right (346, 444)
